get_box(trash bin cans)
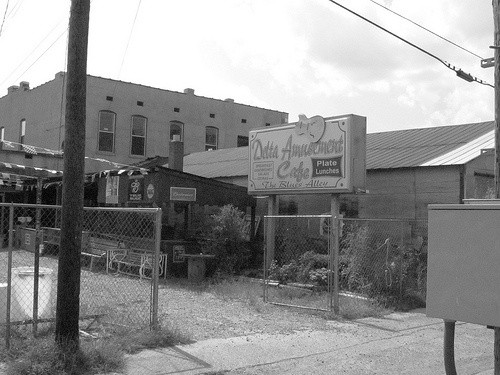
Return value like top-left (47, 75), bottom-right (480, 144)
top-left (161, 240), bottom-right (194, 278)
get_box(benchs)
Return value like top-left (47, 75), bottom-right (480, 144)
top-left (81, 237), bottom-right (123, 272)
top-left (109, 246), bottom-right (165, 280)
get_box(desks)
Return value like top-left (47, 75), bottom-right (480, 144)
top-left (181, 252), bottom-right (218, 282)
